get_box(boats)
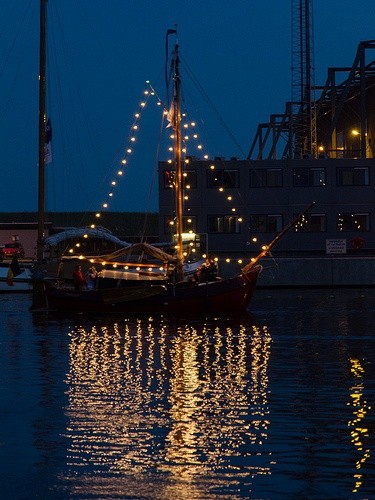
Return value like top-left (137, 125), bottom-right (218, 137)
top-left (0, 265), bottom-right (34, 292)
top-left (41, 226), bottom-right (211, 284)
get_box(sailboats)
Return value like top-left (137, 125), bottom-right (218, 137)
top-left (37, 24), bottom-right (317, 323)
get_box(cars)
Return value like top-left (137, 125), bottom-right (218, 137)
top-left (2, 243), bottom-right (24, 259)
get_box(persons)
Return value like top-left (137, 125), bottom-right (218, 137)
top-left (86, 269), bottom-right (97, 292)
top-left (201, 259), bottom-right (211, 268)
top-left (84, 265), bottom-right (100, 291)
top-left (73, 266), bottom-right (84, 292)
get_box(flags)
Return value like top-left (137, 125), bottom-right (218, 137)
top-left (43, 117), bottom-right (53, 166)
top-left (6, 256), bottom-right (22, 287)
